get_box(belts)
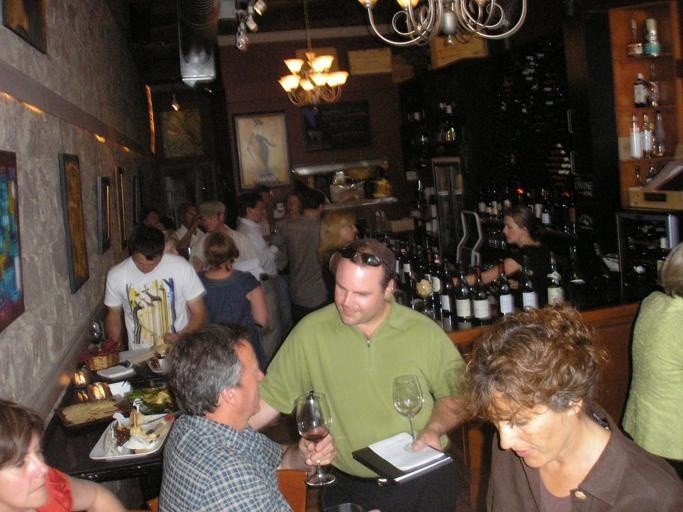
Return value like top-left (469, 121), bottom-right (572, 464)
top-left (340, 472), bottom-right (392, 487)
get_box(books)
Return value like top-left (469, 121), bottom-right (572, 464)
top-left (352, 431), bottom-right (454, 486)
top-left (368, 432), bottom-right (447, 472)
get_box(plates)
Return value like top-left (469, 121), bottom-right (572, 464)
top-left (88, 414), bottom-right (175, 461)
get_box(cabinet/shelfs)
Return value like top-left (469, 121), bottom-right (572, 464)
top-left (610, 0), bottom-right (683, 206)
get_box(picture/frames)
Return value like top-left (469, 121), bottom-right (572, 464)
top-left (57, 150), bottom-right (145, 294)
top-left (233, 110), bottom-right (293, 193)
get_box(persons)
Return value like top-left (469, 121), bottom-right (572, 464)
top-left (246, 118), bottom-right (277, 177)
top-left (1, 400), bottom-right (130, 511)
top-left (454, 304), bottom-right (683, 512)
top-left (160, 324), bottom-right (337, 512)
top-left (449, 204), bottom-right (552, 291)
top-left (99, 184), bottom-right (370, 375)
top-left (243, 237), bottom-right (471, 511)
top-left (620, 239), bottom-right (683, 480)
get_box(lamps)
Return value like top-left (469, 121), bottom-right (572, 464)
top-left (279, 0), bottom-right (349, 109)
top-left (234, 0), bottom-right (267, 51)
top-left (358, 0), bottom-right (528, 50)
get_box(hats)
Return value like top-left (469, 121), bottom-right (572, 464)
top-left (197, 201), bottom-right (225, 216)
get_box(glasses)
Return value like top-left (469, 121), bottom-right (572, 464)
top-left (339, 241), bottom-right (382, 266)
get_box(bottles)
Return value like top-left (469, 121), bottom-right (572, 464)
top-left (494, 259), bottom-right (514, 319)
top-left (382, 235), bottom-right (492, 326)
top-left (520, 264), bottom-right (538, 313)
top-left (477, 186), bottom-right (576, 234)
top-left (544, 252), bottom-right (563, 307)
top-left (625, 18), bottom-right (666, 208)
top-left (655, 235), bottom-right (669, 283)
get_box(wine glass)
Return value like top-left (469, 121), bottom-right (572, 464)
top-left (87, 318), bottom-right (104, 359)
top-left (391, 375), bottom-right (424, 450)
top-left (295, 393), bottom-right (336, 486)
